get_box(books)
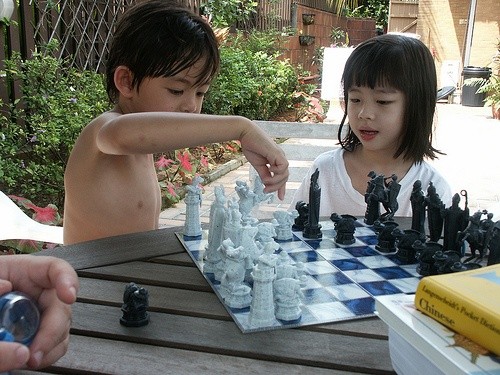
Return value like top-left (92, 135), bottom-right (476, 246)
top-left (367, 294), bottom-right (499, 374)
top-left (415, 263), bottom-right (500, 356)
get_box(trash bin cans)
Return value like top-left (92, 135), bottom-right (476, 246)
top-left (461, 66), bottom-right (492, 107)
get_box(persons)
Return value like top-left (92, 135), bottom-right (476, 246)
top-left (182, 161), bottom-right (303, 290)
top-left (285, 34), bottom-right (452, 218)
top-left (307, 167), bottom-right (321, 226)
top-left (62, 1), bottom-right (291, 246)
top-left (364, 171), bottom-right (500, 267)
top-left (0, 253), bottom-right (81, 371)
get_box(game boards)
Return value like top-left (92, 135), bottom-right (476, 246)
top-left (176, 217), bottom-right (459, 332)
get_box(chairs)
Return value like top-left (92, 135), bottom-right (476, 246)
top-left (1, 191), bottom-right (63, 245)
top-left (248, 120), bottom-right (352, 216)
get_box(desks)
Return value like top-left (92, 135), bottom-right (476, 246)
top-left (0, 218), bottom-right (500, 375)
